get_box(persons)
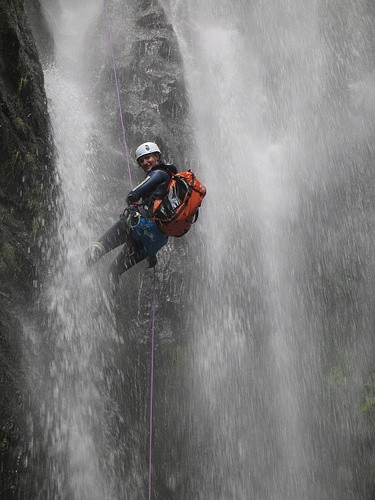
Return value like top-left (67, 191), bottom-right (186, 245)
top-left (84, 142), bottom-right (179, 290)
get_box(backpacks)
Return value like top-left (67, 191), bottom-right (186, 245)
top-left (143, 168), bottom-right (208, 238)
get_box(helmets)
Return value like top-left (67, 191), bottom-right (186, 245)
top-left (135, 141), bottom-right (161, 160)
